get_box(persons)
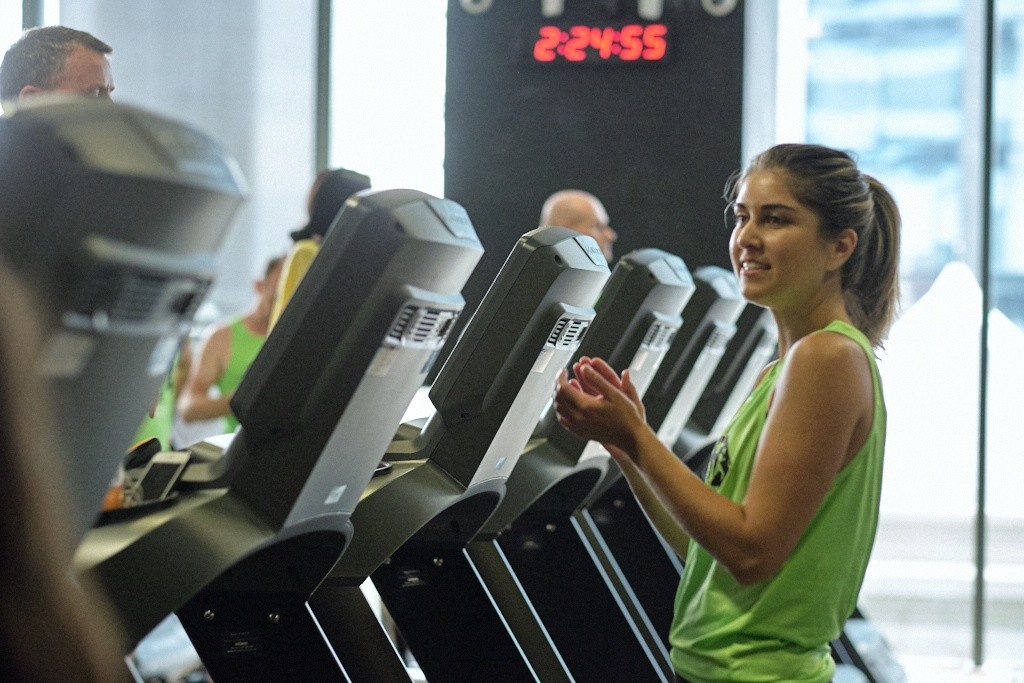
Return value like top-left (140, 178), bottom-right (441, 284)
top-left (541, 189), bottom-right (618, 266)
top-left (271, 166), bottom-right (372, 327)
top-left (128, 335), bottom-right (193, 453)
top-left (0, 24), bottom-right (117, 116)
top-left (185, 256), bottom-right (284, 433)
top-left (553, 145), bottom-right (902, 683)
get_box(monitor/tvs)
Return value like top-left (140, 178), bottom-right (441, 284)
top-left (224, 188), bottom-right (781, 538)
top-left (0, 96), bottom-right (245, 565)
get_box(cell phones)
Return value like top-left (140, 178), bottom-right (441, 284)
top-left (126, 451), bottom-right (193, 502)
